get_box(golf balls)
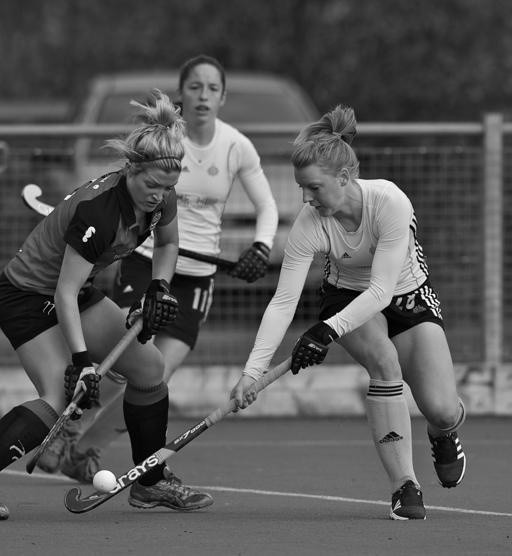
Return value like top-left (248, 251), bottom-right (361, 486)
top-left (91, 468), bottom-right (118, 495)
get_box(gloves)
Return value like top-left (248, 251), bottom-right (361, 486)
top-left (63, 349), bottom-right (103, 421)
top-left (230, 240), bottom-right (272, 283)
top-left (290, 319), bottom-right (340, 376)
top-left (124, 276), bottom-right (181, 345)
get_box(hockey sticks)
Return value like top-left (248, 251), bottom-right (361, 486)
top-left (20, 182), bottom-right (267, 280)
top-left (25, 313), bottom-right (143, 475)
top-left (64, 350), bottom-right (294, 515)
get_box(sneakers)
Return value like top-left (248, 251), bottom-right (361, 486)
top-left (127, 464), bottom-right (215, 512)
top-left (389, 478), bottom-right (428, 521)
top-left (36, 423), bottom-right (102, 485)
top-left (425, 422), bottom-right (468, 489)
top-left (0, 501), bottom-right (10, 521)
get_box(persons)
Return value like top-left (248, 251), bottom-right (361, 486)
top-left (40, 53), bottom-right (281, 485)
top-left (234, 102), bottom-right (465, 521)
top-left (1, 86), bottom-right (213, 519)
